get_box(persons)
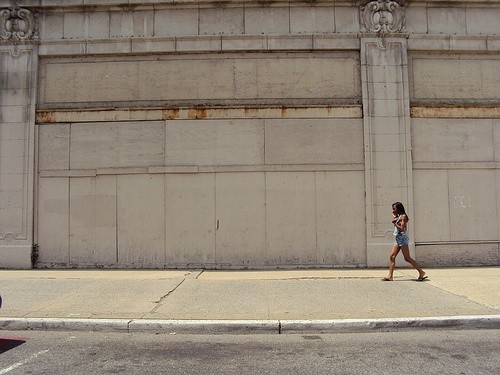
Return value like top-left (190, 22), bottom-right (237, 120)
top-left (383, 202), bottom-right (428, 282)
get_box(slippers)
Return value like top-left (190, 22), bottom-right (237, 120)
top-left (416, 275), bottom-right (428, 281)
top-left (381, 277), bottom-right (392, 280)
